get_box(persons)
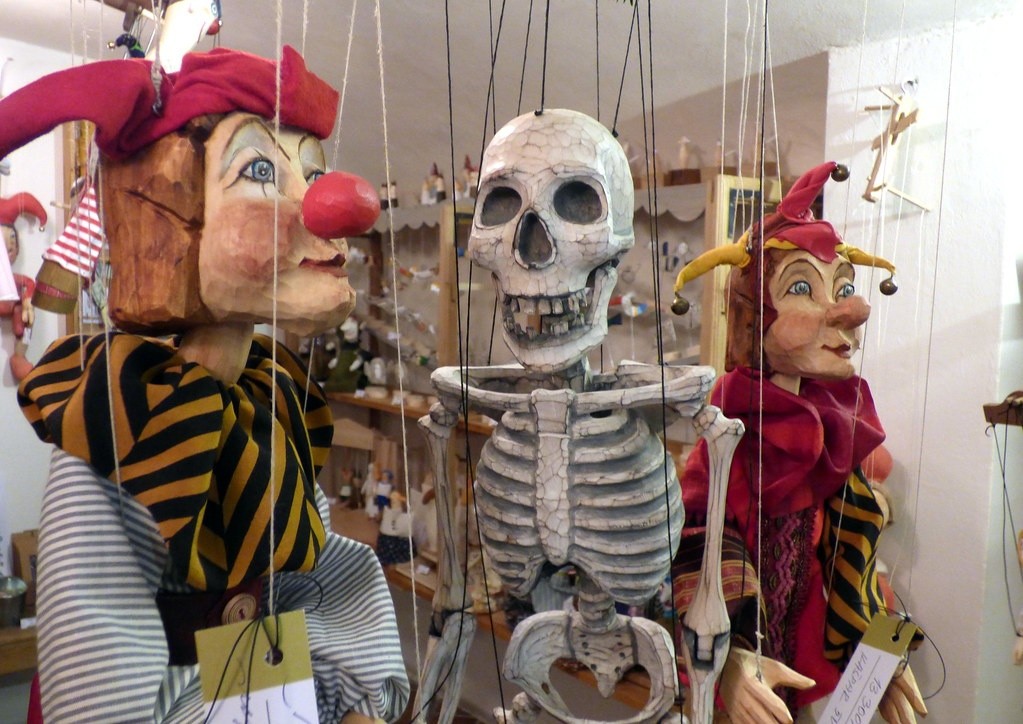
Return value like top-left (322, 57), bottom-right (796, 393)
top-left (0, 46), bottom-right (409, 724)
top-left (0, 193), bottom-right (47, 381)
top-left (326, 314), bottom-right (370, 398)
top-left (670, 161), bottom-right (928, 724)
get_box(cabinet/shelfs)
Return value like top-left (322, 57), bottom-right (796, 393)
top-left (288, 166), bottom-right (822, 724)
